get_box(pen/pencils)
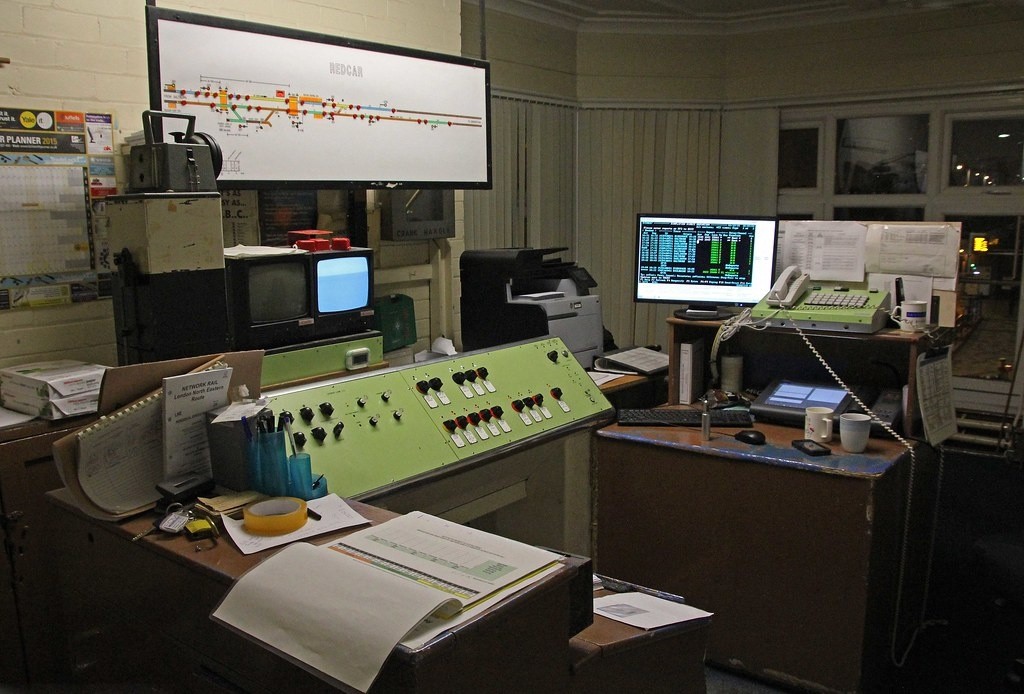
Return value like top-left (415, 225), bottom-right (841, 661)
top-left (242, 412), bottom-right (287, 433)
top-left (286, 424), bottom-right (298, 458)
top-left (308, 510), bottom-right (322, 521)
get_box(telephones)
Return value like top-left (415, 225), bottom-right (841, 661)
top-left (765, 264), bottom-right (810, 306)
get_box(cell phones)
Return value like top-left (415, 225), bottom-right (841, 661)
top-left (792, 439), bottom-right (832, 457)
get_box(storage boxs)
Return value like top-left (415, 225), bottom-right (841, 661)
top-left (105, 193), bottom-right (226, 274)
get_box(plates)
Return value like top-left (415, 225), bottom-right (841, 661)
top-left (311, 474), bottom-right (328, 498)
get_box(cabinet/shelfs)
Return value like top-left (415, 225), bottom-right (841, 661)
top-left (1, 308), bottom-right (945, 694)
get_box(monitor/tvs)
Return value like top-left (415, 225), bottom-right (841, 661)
top-left (749, 379), bottom-right (858, 429)
top-left (224, 246), bottom-right (376, 352)
top-left (634, 213), bottom-right (779, 321)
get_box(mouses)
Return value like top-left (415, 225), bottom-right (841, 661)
top-left (734, 430), bottom-right (766, 445)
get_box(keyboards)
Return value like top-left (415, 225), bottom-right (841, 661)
top-left (617, 408), bottom-right (752, 428)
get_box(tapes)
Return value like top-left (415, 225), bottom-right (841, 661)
top-left (243, 495), bottom-right (308, 535)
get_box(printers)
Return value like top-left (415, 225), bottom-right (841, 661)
top-left (460, 246), bottom-right (603, 370)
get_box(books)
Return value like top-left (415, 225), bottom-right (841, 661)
top-left (51, 354), bottom-right (234, 522)
top-left (209, 510), bottom-right (568, 694)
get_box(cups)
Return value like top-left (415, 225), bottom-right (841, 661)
top-left (290, 453), bottom-right (313, 500)
top-left (249, 428), bottom-right (289, 498)
top-left (805, 407), bottom-right (834, 443)
top-left (840, 413), bottom-right (871, 453)
top-left (890, 301), bottom-right (927, 333)
